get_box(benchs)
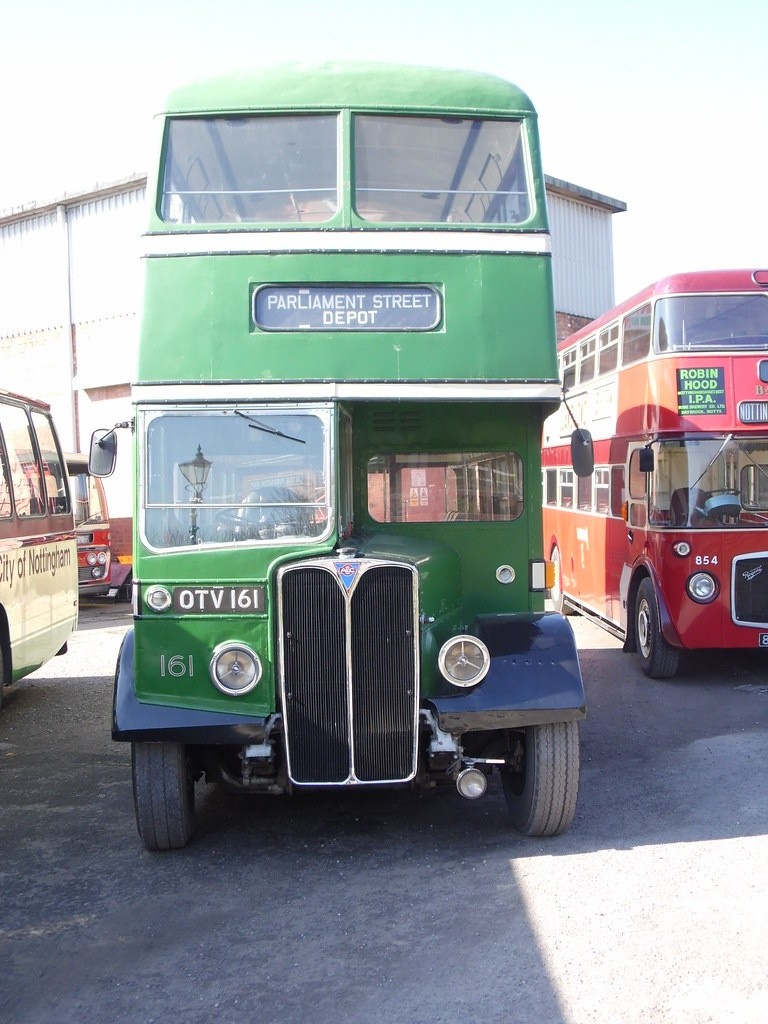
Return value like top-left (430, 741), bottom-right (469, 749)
top-left (689, 328), bottom-right (768, 349)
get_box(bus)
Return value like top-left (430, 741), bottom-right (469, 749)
top-left (545, 268), bottom-right (768, 680)
top-left (88, 59), bottom-right (594, 851)
top-left (18, 453), bottom-right (113, 597)
top-left (0, 388), bottom-right (79, 710)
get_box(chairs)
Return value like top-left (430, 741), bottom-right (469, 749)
top-left (670, 487), bottom-right (716, 525)
top-left (232, 485), bottom-right (311, 542)
